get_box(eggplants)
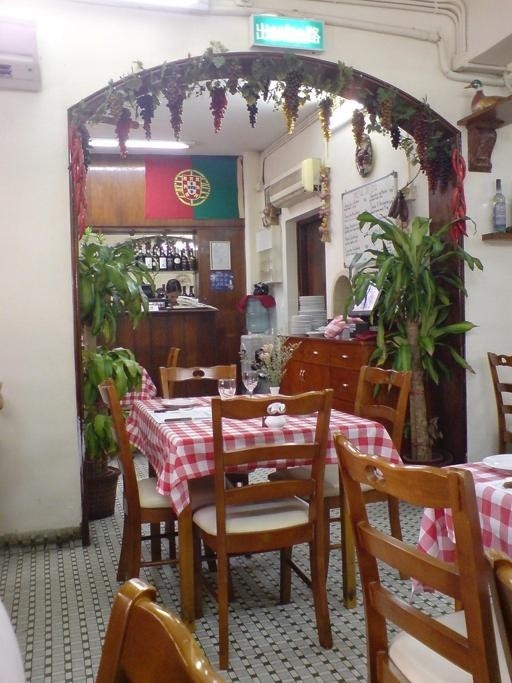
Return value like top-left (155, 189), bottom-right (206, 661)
top-left (393, 194), bottom-right (400, 218)
top-left (400, 193), bottom-right (409, 221)
top-left (388, 191), bottom-right (398, 217)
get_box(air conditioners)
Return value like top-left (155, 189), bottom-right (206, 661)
top-left (266, 156), bottom-right (318, 209)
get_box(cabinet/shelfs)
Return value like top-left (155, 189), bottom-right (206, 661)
top-left (93, 303), bottom-right (218, 399)
top-left (74, 219), bottom-right (247, 364)
top-left (279, 335), bottom-right (437, 461)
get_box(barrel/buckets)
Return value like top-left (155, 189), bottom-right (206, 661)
top-left (246, 298), bottom-right (268, 334)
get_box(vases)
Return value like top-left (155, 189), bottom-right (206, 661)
top-left (264, 385), bottom-right (288, 426)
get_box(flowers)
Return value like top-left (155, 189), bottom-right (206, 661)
top-left (238, 329), bottom-right (302, 384)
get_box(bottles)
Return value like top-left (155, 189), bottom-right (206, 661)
top-left (134, 243), bottom-right (146, 267)
top-left (145, 242), bottom-right (152, 271)
top-left (492, 179), bottom-right (505, 233)
top-left (188, 249), bottom-right (196, 271)
top-left (174, 247), bottom-right (181, 271)
top-left (167, 248), bottom-right (174, 271)
top-left (152, 248), bottom-right (159, 271)
top-left (162, 284), bottom-right (166, 291)
top-left (188, 286), bottom-right (195, 296)
top-left (181, 248), bottom-right (188, 271)
top-left (182, 286), bottom-right (187, 297)
top-left (159, 247), bottom-right (167, 271)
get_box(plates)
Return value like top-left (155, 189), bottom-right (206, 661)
top-left (481, 453), bottom-right (512, 477)
top-left (290, 296), bottom-right (326, 337)
top-left (161, 398), bottom-right (199, 409)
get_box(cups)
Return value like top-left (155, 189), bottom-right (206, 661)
top-left (217, 378), bottom-right (237, 400)
top-left (241, 369), bottom-right (259, 399)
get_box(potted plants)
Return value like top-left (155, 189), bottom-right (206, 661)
top-left (345, 211), bottom-right (483, 467)
top-left (76, 226), bottom-right (157, 520)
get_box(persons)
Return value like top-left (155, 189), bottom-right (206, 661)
top-left (165, 279), bottom-right (182, 307)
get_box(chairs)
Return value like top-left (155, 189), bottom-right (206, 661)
top-left (157, 346), bottom-right (180, 398)
top-left (93, 577), bottom-right (219, 683)
top-left (193, 389), bottom-right (335, 669)
top-left (335, 433), bottom-right (499, 683)
top-left (104, 379), bottom-right (235, 600)
top-left (488, 350), bottom-right (512, 454)
top-left (281, 366), bottom-right (418, 603)
top-left (159, 365), bottom-right (256, 567)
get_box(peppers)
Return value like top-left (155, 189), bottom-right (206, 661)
top-left (68, 124), bottom-right (87, 182)
top-left (451, 148), bottom-right (458, 175)
top-left (75, 182), bottom-right (80, 212)
top-left (459, 206), bottom-right (466, 231)
top-left (81, 186), bottom-right (87, 209)
top-left (455, 183), bottom-right (462, 192)
top-left (452, 188), bottom-right (458, 214)
top-left (452, 223), bottom-right (459, 238)
top-left (458, 155), bottom-right (467, 180)
top-left (78, 209), bottom-right (86, 239)
top-left (460, 191), bottom-right (465, 213)
top-left (457, 221), bottom-right (469, 238)
top-left (450, 228), bottom-right (456, 241)
